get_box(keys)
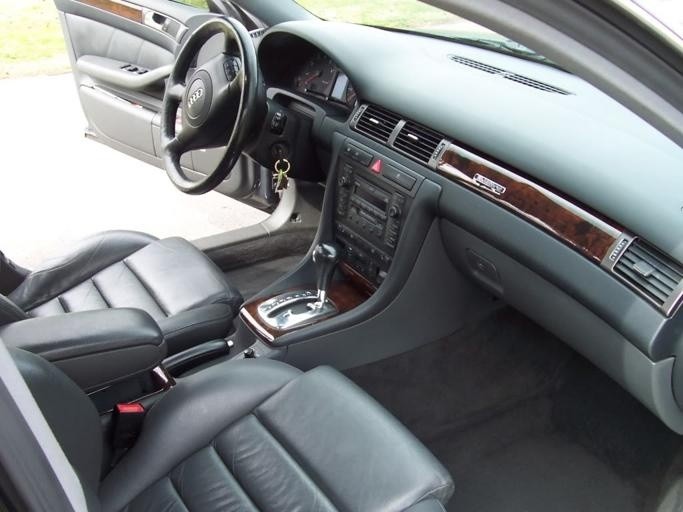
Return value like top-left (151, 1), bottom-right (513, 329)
top-left (271, 147), bottom-right (291, 200)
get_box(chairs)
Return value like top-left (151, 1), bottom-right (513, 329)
top-left (0, 230), bottom-right (242, 359)
top-left (0, 341), bottom-right (454, 512)
